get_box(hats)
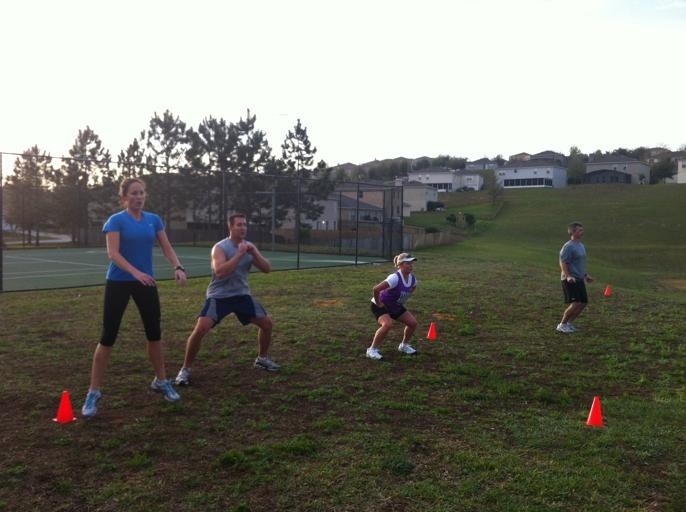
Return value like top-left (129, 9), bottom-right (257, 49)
top-left (397, 254), bottom-right (418, 263)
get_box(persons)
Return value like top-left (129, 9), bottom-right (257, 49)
top-left (80, 177), bottom-right (188, 419)
top-left (364, 252), bottom-right (422, 361)
top-left (556, 219), bottom-right (596, 335)
top-left (173, 212), bottom-right (284, 386)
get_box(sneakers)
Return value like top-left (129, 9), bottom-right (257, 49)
top-left (82, 389), bottom-right (101, 416)
top-left (366, 347), bottom-right (383, 360)
top-left (175, 367), bottom-right (192, 385)
top-left (150, 376), bottom-right (181, 402)
top-left (556, 324), bottom-right (575, 333)
top-left (254, 357), bottom-right (281, 370)
top-left (398, 343), bottom-right (417, 354)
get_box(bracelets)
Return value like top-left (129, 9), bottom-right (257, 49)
top-left (173, 263), bottom-right (186, 271)
top-left (583, 271), bottom-right (588, 276)
